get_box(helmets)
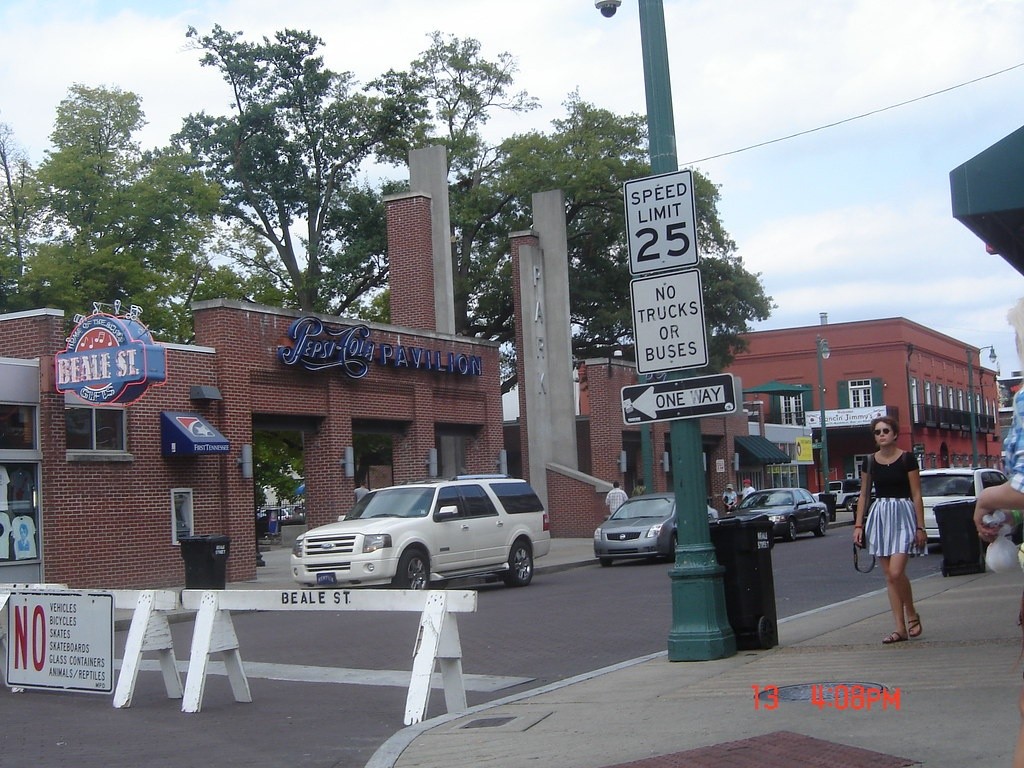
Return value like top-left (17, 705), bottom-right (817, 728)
top-left (995, 358), bottom-right (1024, 381)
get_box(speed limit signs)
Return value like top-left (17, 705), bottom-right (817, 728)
top-left (623, 169), bottom-right (699, 276)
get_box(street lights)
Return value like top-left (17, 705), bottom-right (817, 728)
top-left (815, 334), bottom-right (831, 495)
top-left (966, 345), bottom-right (998, 469)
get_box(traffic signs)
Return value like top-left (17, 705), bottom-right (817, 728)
top-left (621, 372), bottom-right (737, 426)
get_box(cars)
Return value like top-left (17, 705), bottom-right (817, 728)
top-left (709, 487), bottom-right (829, 541)
top-left (919, 467), bottom-right (1010, 547)
top-left (593, 491), bottom-right (719, 567)
top-left (256, 506), bottom-right (305, 520)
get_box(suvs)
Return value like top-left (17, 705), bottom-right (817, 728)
top-left (289, 473), bottom-right (551, 590)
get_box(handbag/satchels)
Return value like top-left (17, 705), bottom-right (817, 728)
top-left (857, 498), bottom-right (876, 548)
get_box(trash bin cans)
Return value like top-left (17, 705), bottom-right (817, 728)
top-left (178, 534), bottom-right (229, 590)
top-left (819, 493), bottom-right (836, 522)
top-left (709, 513), bottom-right (779, 649)
top-left (934, 498), bottom-right (985, 577)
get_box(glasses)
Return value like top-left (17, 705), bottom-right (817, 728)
top-left (874, 428), bottom-right (893, 435)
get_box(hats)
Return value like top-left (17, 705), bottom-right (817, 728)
top-left (743, 479), bottom-right (751, 483)
top-left (727, 484), bottom-right (734, 490)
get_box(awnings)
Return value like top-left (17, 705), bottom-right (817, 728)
top-left (734, 435), bottom-right (791, 465)
top-left (161, 411), bottom-right (230, 456)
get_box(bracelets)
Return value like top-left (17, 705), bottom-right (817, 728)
top-left (917, 527), bottom-right (926, 531)
top-left (1012, 510), bottom-right (1021, 525)
top-left (855, 526), bottom-right (863, 528)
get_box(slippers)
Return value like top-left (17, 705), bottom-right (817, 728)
top-left (907, 613), bottom-right (922, 637)
top-left (882, 632), bottom-right (908, 644)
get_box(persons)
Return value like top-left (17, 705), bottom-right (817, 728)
top-left (742, 479), bottom-right (755, 507)
top-left (606, 482), bottom-right (628, 515)
top-left (974, 292), bottom-right (1024, 768)
top-left (18, 524), bottom-right (29, 551)
top-left (354, 480), bottom-right (369, 504)
top-left (722, 484), bottom-right (737, 513)
top-left (853, 417), bottom-right (928, 643)
top-left (292, 507), bottom-right (304, 521)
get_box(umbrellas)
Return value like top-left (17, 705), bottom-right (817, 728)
top-left (294, 483), bottom-right (305, 495)
top-left (742, 380), bottom-right (813, 423)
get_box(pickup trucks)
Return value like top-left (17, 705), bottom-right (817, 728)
top-left (811, 481), bottom-right (875, 512)
